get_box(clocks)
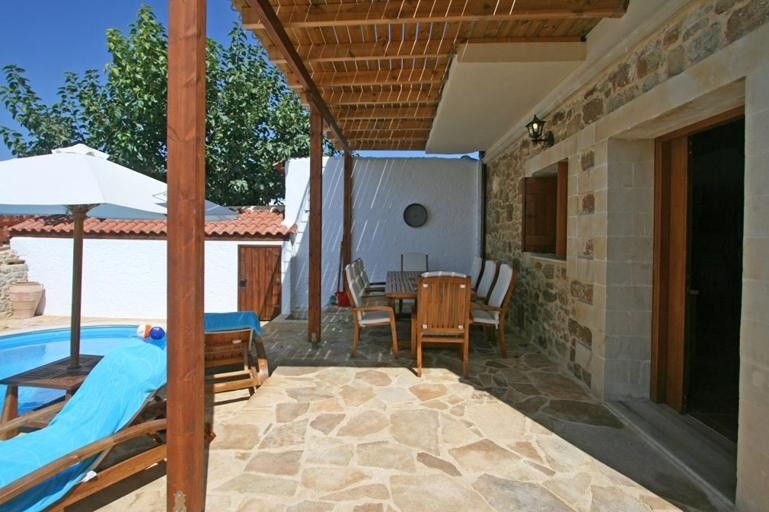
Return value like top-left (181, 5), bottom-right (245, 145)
top-left (403, 203), bottom-right (427, 227)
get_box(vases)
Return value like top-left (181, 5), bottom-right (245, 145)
top-left (8, 282), bottom-right (45, 319)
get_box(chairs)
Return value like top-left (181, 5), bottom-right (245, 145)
top-left (400, 253), bottom-right (429, 318)
top-left (411, 271), bottom-right (471, 379)
top-left (344, 258), bottom-right (399, 358)
top-left (0, 337), bottom-right (217, 512)
top-left (470, 256), bottom-right (521, 357)
top-left (204, 311), bottom-right (269, 397)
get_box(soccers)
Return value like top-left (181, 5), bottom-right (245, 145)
top-left (150, 327), bottom-right (165, 340)
top-left (136, 324), bottom-right (152, 338)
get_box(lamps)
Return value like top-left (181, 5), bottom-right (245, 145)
top-left (525, 114), bottom-right (554, 145)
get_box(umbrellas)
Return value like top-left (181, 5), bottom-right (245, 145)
top-left (0, 143), bottom-right (239, 369)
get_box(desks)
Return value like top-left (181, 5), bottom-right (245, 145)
top-left (0, 355), bottom-right (105, 442)
